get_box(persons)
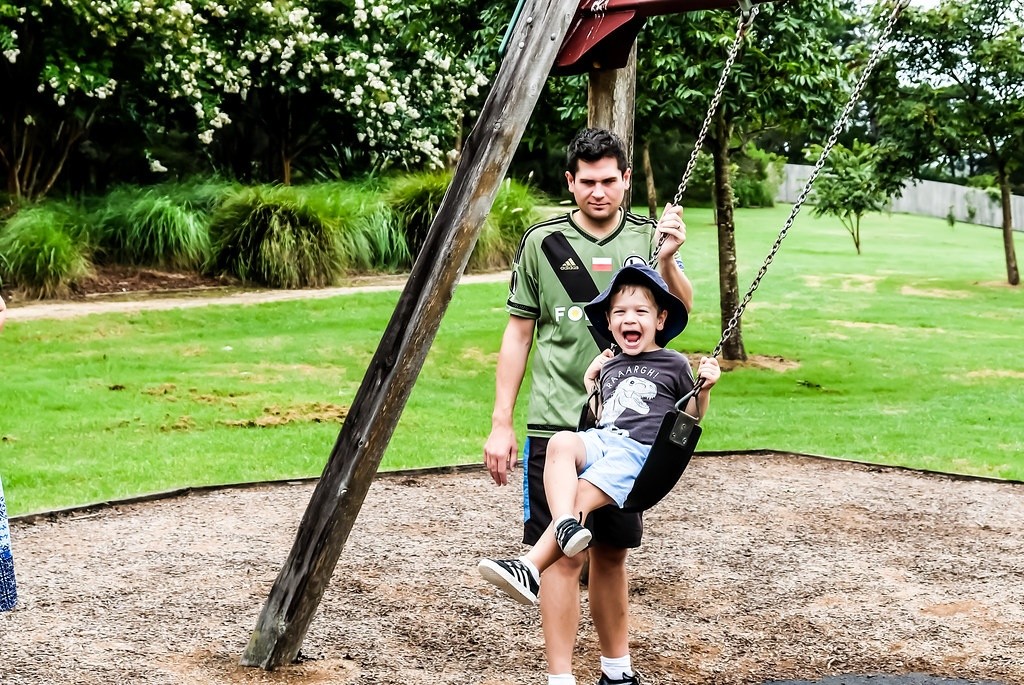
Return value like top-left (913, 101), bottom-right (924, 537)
top-left (484, 128), bottom-right (693, 685)
top-left (477, 263), bottom-right (720, 604)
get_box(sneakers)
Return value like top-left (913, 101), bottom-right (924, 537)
top-left (598, 672), bottom-right (641, 685)
top-left (555, 511), bottom-right (592, 558)
top-left (477, 556), bottom-right (540, 606)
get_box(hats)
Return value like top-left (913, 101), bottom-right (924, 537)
top-left (582, 263), bottom-right (690, 350)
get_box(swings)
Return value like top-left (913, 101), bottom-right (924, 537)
top-left (569, 2), bottom-right (904, 515)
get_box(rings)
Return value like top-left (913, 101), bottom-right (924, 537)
top-left (677, 225), bottom-right (680, 229)
